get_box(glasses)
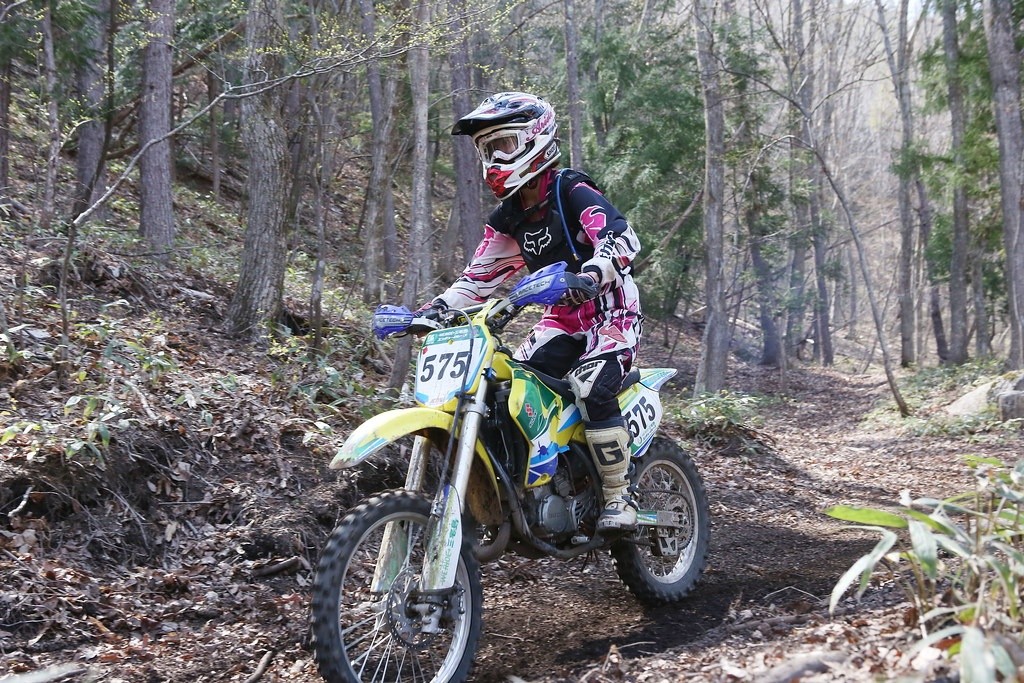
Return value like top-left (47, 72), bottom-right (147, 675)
top-left (472, 105), bottom-right (556, 164)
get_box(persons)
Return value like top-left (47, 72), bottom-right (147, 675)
top-left (404, 91), bottom-right (644, 535)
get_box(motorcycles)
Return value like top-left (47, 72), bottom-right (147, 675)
top-left (304, 261), bottom-right (714, 683)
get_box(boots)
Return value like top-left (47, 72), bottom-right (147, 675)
top-left (582, 416), bottom-right (639, 535)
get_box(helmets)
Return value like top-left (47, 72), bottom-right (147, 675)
top-left (451, 92), bottom-right (562, 200)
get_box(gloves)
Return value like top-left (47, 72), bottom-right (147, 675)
top-left (560, 272), bottom-right (596, 306)
top-left (405, 302), bottom-right (445, 334)
